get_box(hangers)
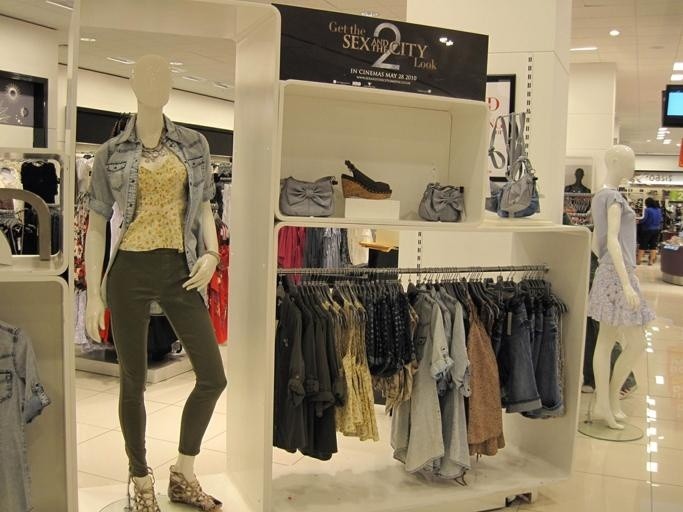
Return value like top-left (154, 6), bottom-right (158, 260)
top-left (280, 263), bottom-right (568, 321)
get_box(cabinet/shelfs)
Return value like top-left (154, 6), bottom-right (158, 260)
top-left (619, 185), bottom-right (683, 232)
top-left (1, 0), bottom-right (79, 512)
top-left (228, 2), bottom-right (591, 512)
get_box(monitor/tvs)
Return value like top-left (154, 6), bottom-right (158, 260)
top-left (663, 85), bottom-right (683, 124)
top-left (662, 90), bottom-right (683, 128)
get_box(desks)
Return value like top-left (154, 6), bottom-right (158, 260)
top-left (661, 244), bottom-right (683, 286)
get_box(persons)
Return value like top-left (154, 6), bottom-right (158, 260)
top-left (85, 54), bottom-right (227, 512)
top-left (636, 198), bottom-right (659, 265)
top-left (564, 168), bottom-right (591, 213)
top-left (592, 144), bottom-right (657, 428)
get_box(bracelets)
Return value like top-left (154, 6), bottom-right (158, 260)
top-left (200, 251), bottom-right (222, 265)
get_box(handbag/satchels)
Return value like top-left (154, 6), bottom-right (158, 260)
top-left (279, 176), bottom-right (338, 216)
top-left (418, 183), bottom-right (464, 222)
top-left (498, 169), bottom-right (540, 217)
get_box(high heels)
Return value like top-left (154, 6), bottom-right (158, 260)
top-left (341, 161), bottom-right (392, 199)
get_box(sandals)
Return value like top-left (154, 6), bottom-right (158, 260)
top-left (168, 465), bottom-right (222, 512)
top-left (128, 467), bottom-right (160, 512)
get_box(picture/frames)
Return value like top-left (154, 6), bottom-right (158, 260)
top-left (485, 74), bottom-right (516, 182)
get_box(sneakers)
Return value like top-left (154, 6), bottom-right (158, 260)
top-left (619, 384), bottom-right (637, 400)
top-left (581, 385), bottom-right (594, 393)
